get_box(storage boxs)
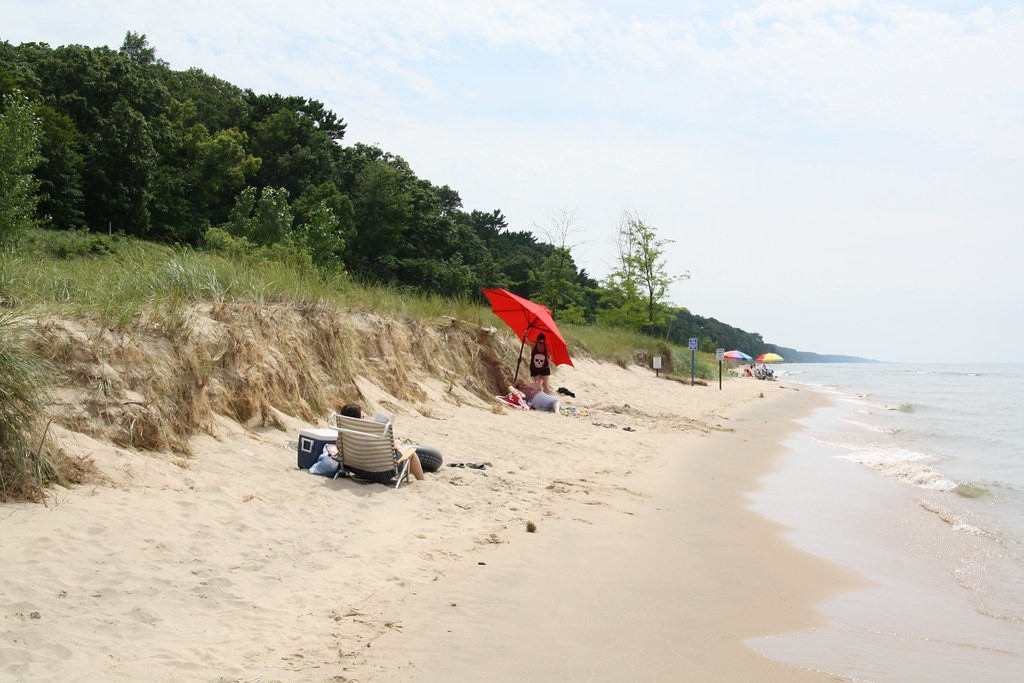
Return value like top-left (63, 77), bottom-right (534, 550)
top-left (297, 429), bottom-right (338, 469)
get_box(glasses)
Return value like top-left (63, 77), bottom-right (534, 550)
top-left (538, 341), bottom-right (544, 344)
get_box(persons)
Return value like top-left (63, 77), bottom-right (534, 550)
top-left (742, 363), bottom-right (773, 381)
top-left (516, 378), bottom-right (561, 414)
top-left (523, 330), bottom-right (551, 392)
top-left (339, 404), bottom-right (427, 480)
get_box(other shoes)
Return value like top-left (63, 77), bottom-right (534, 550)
top-left (466, 463), bottom-right (485, 469)
top-left (447, 463), bottom-right (465, 468)
top-left (592, 422), bottom-right (601, 426)
top-left (623, 427), bottom-right (637, 432)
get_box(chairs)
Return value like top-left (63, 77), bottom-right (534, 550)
top-left (327, 411), bottom-right (416, 490)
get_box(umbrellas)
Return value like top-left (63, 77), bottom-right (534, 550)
top-left (723, 349), bottom-right (753, 366)
top-left (482, 286), bottom-right (575, 383)
top-left (756, 352), bottom-right (784, 365)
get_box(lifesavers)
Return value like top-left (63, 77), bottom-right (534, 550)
top-left (412, 445), bottom-right (443, 471)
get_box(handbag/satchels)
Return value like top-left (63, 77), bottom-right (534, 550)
top-left (309, 443), bottom-right (341, 476)
